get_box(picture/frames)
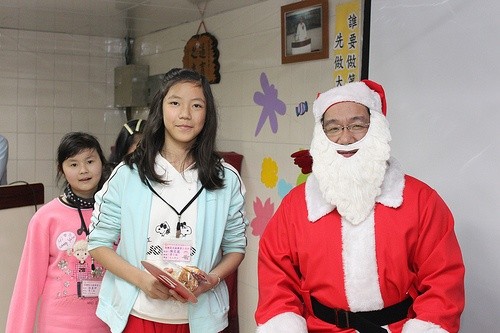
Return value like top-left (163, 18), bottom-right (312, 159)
top-left (279, 0), bottom-right (331, 65)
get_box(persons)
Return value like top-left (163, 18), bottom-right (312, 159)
top-left (87, 68), bottom-right (247, 333)
top-left (5, 132), bottom-right (121, 332)
top-left (254, 80), bottom-right (466, 333)
top-left (295, 15), bottom-right (307, 41)
top-left (108, 120), bottom-right (148, 163)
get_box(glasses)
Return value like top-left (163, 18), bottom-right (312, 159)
top-left (323, 122), bottom-right (370, 135)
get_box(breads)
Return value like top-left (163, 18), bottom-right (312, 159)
top-left (177, 267), bottom-right (206, 294)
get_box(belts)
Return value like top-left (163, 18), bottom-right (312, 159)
top-left (310, 296), bottom-right (413, 333)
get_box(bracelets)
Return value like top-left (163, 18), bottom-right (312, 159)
top-left (208, 273), bottom-right (221, 291)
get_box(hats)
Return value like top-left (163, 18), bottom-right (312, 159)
top-left (313, 79), bottom-right (386, 122)
